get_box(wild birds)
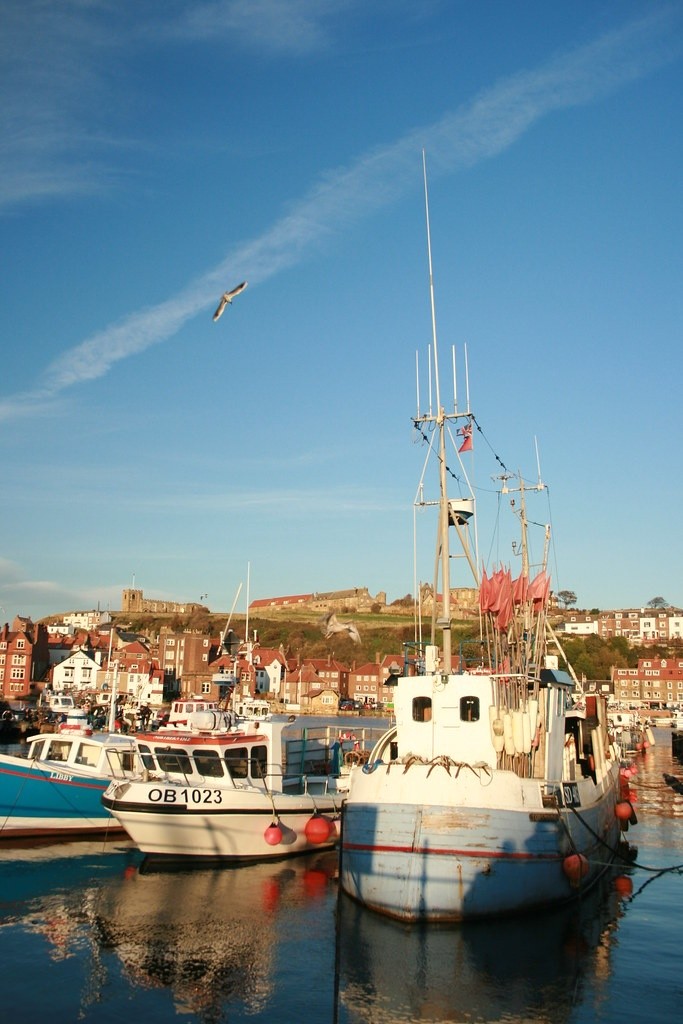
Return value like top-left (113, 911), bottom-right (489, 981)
top-left (212, 279), bottom-right (249, 321)
top-left (200, 592), bottom-right (209, 601)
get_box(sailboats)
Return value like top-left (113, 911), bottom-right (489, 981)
top-left (337, 147), bottom-right (634, 926)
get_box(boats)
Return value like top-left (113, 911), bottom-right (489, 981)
top-left (99, 562), bottom-right (371, 864)
top-left (0, 658), bottom-right (138, 837)
